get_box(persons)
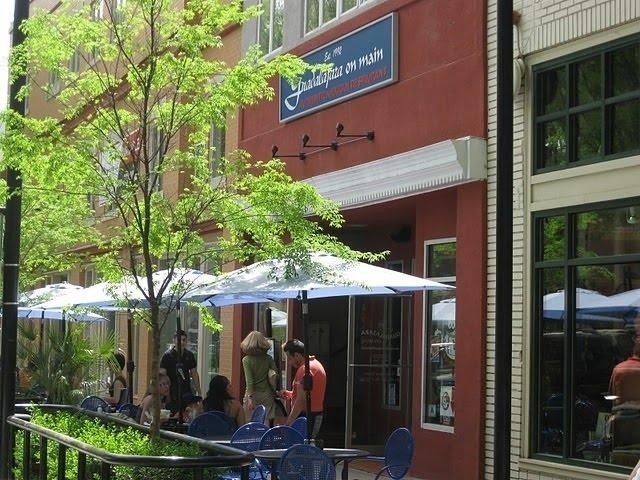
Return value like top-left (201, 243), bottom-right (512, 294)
top-left (556, 332), bottom-right (593, 380)
top-left (240, 329), bottom-right (326, 442)
top-left (608, 340), bottom-right (640, 411)
top-left (99, 350), bottom-right (128, 413)
top-left (545, 361), bottom-right (593, 415)
top-left (15, 330), bottom-right (42, 392)
top-left (139, 329), bottom-right (245, 429)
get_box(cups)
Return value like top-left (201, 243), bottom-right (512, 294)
top-left (162, 410), bottom-right (170, 417)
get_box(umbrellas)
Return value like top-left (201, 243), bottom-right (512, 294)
top-left (177, 248), bottom-right (454, 448)
top-left (27, 283), bottom-right (134, 313)
top-left (431, 287), bottom-right (639, 323)
top-left (77, 269), bottom-right (275, 343)
top-left (267, 305), bottom-right (287, 327)
top-left (20, 305), bottom-right (105, 321)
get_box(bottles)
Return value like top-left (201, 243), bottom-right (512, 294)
top-left (97, 405), bottom-right (103, 413)
top-left (304, 439), bottom-right (316, 454)
top-left (110, 404), bottom-right (116, 413)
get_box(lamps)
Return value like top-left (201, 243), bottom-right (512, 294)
top-left (270, 145), bottom-right (307, 163)
top-left (302, 134), bottom-right (340, 153)
top-left (334, 122), bottom-right (376, 141)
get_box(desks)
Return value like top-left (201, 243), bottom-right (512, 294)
top-left (250, 448), bottom-right (371, 478)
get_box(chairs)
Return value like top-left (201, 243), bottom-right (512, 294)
top-left (255, 425), bottom-right (306, 480)
top-left (335, 428), bottom-right (414, 480)
top-left (78, 393), bottom-right (308, 449)
top-left (540, 392), bottom-right (640, 467)
top-left (223, 422), bottom-right (273, 479)
top-left (277, 444), bottom-right (336, 480)
